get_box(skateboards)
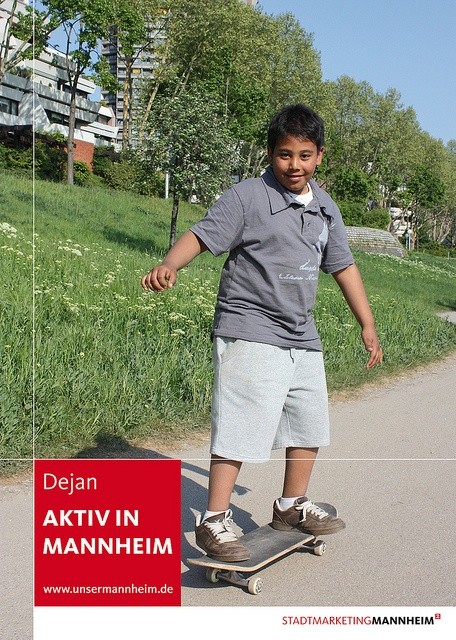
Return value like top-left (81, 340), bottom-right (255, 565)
top-left (187, 501), bottom-right (337, 594)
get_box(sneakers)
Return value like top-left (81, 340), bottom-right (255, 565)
top-left (271, 497), bottom-right (344, 535)
top-left (194, 509), bottom-right (250, 562)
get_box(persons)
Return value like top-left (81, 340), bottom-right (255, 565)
top-left (140, 102), bottom-right (383, 562)
